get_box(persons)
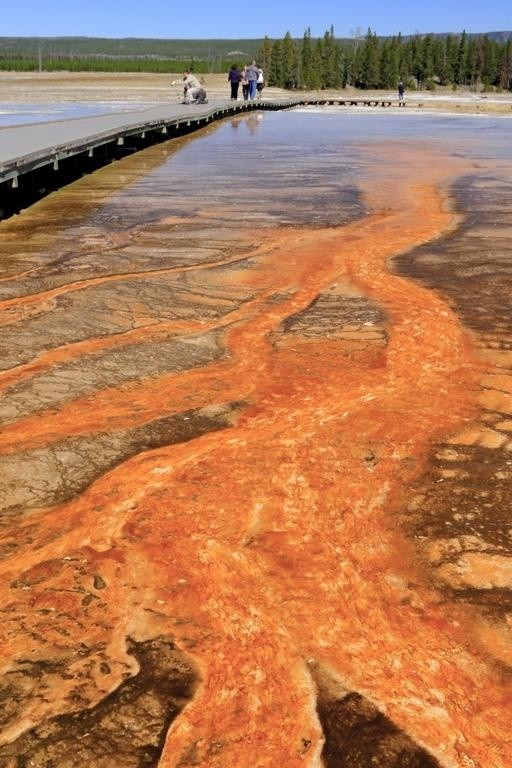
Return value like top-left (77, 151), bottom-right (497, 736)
top-left (256, 65), bottom-right (264, 99)
top-left (240, 66), bottom-right (249, 100)
top-left (246, 59), bottom-right (259, 99)
top-left (227, 63), bottom-right (242, 101)
top-left (398, 81), bottom-right (403, 100)
top-left (181, 75), bottom-right (198, 104)
top-left (174, 70), bottom-right (207, 105)
